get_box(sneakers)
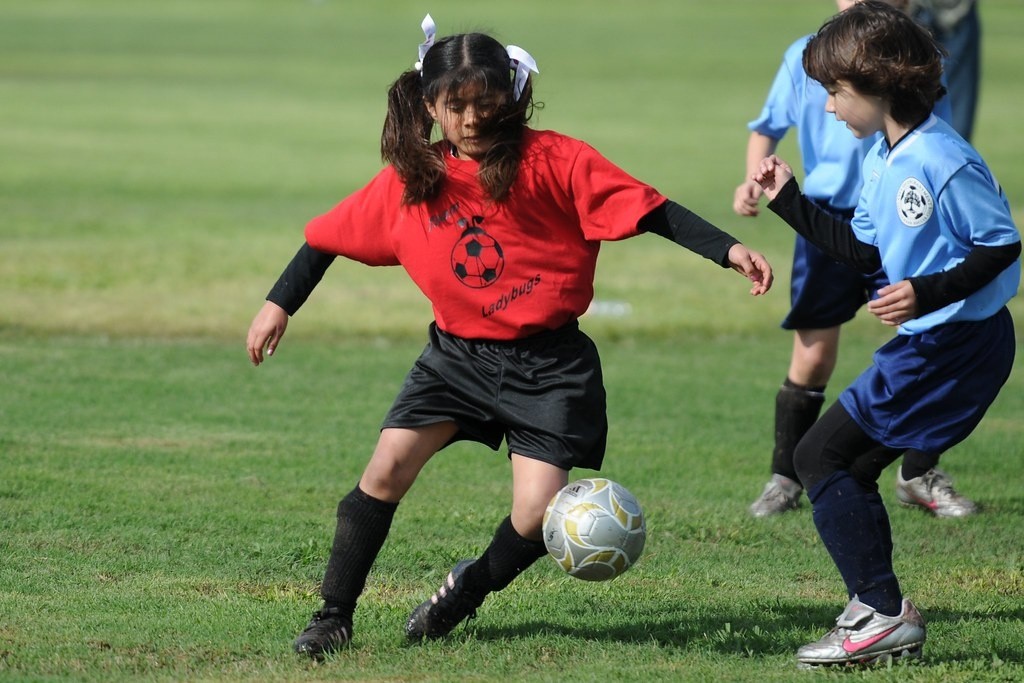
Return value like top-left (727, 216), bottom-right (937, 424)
top-left (404, 559), bottom-right (484, 642)
top-left (294, 607), bottom-right (353, 659)
top-left (750, 480), bottom-right (804, 519)
top-left (797, 593), bottom-right (926, 663)
top-left (896, 465), bottom-right (983, 519)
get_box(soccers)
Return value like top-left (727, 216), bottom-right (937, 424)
top-left (542, 477), bottom-right (645, 582)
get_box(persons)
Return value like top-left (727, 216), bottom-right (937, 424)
top-left (749, 1), bottom-right (1021, 665)
top-left (894, 0), bottom-right (981, 142)
top-left (736, 1), bottom-right (981, 522)
top-left (246, 13), bottom-right (773, 670)
